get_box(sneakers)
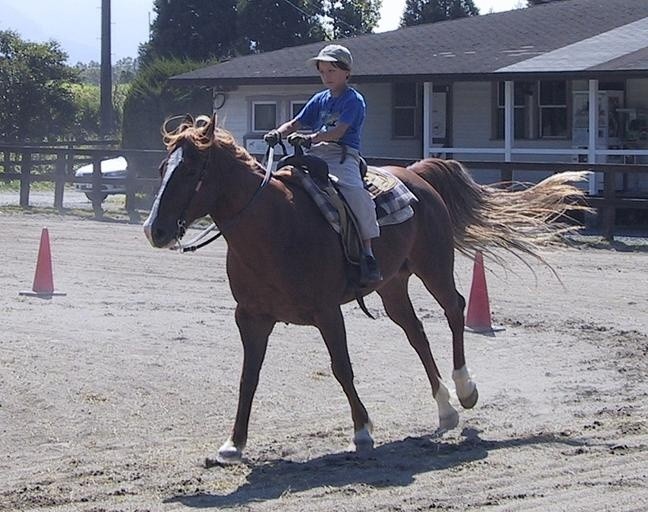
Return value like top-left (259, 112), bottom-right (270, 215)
top-left (360, 256), bottom-right (381, 288)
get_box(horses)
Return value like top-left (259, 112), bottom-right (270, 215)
top-left (137, 110), bottom-right (598, 467)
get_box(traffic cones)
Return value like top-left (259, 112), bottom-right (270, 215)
top-left (464, 250), bottom-right (505, 333)
top-left (20, 227), bottom-right (67, 296)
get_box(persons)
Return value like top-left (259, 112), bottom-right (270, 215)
top-left (264, 44), bottom-right (381, 283)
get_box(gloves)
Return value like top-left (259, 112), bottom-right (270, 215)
top-left (265, 130), bottom-right (282, 146)
top-left (288, 133), bottom-right (311, 149)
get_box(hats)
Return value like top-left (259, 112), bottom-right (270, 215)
top-left (305, 44), bottom-right (353, 69)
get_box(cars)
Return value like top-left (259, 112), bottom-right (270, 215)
top-left (73, 156), bottom-right (127, 200)
